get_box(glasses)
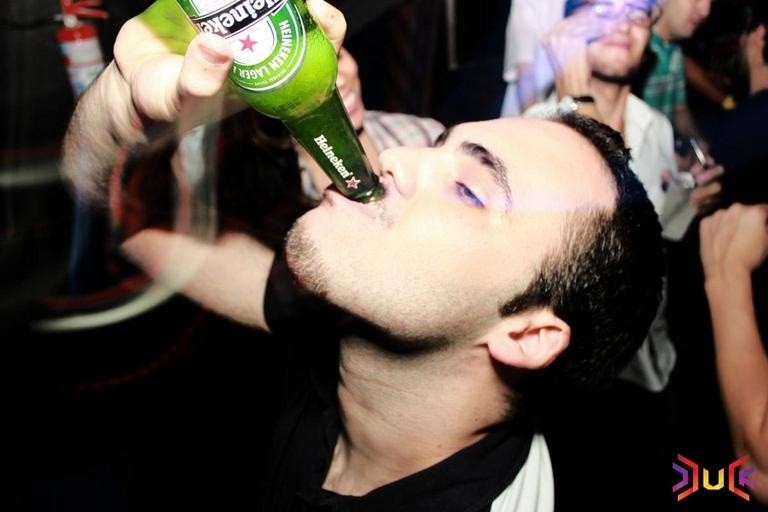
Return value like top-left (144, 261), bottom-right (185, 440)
top-left (589, 0), bottom-right (652, 30)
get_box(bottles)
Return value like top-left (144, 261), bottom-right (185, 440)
top-left (177, 0), bottom-right (386, 204)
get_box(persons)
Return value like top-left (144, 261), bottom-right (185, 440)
top-left (0, 2), bottom-right (766, 510)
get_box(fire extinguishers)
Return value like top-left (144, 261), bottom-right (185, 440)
top-left (54, 0), bottom-right (107, 100)
